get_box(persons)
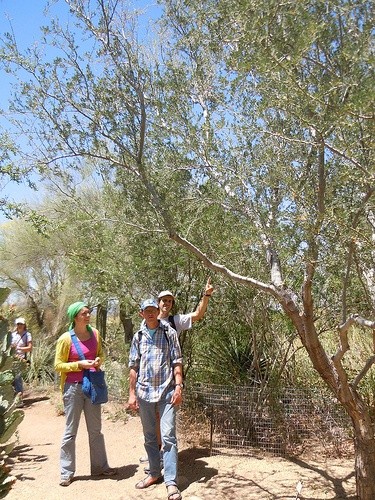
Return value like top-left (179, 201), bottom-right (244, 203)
top-left (138, 276), bottom-right (215, 474)
top-left (126, 298), bottom-right (188, 500)
top-left (8, 317), bottom-right (33, 407)
top-left (52, 300), bottom-right (119, 485)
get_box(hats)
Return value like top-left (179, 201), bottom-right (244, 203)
top-left (141, 299), bottom-right (159, 310)
top-left (15, 318), bottom-right (26, 325)
top-left (158, 290), bottom-right (174, 299)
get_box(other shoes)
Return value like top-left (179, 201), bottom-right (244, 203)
top-left (139, 456), bottom-right (150, 463)
top-left (91, 471), bottom-right (121, 480)
top-left (59, 479), bottom-right (71, 485)
top-left (144, 463), bottom-right (164, 473)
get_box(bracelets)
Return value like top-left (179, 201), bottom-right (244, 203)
top-left (203, 294), bottom-right (211, 297)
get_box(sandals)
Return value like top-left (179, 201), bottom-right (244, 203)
top-left (135, 474), bottom-right (162, 489)
top-left (166, 485), bottom-right (181, 500)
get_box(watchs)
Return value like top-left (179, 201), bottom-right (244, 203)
top-left (174, 384), bottom-right (184, 388)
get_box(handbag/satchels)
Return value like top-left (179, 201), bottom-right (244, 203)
top-left (81, 369), bottom-right (108, 404)
